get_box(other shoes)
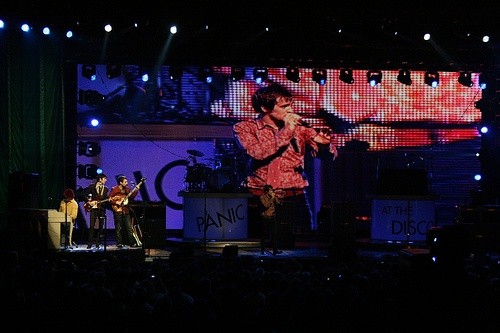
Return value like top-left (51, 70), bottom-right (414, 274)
top-left (59, 239), bottom-right (143, 250)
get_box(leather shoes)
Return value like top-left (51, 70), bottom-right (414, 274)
top-left (273, 248), bottom-right (283, 255)
top-left (260, 248), bottom-right (267, 253)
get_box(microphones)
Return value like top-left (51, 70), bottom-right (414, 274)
top-left (297, 119), bottom-right (311, 128)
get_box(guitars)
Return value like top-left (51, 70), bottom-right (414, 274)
top-left (112, 177), bottom-right (147, 211)
top-left (84, 195), bottom-right (121, 211)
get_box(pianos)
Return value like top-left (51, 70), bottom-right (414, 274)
top-left (18, 207), bottom-right (73, 249)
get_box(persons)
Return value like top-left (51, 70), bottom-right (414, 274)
top-left (57, 188), bottom-right (79, 247)
top-left (231, 82), bottom-right (341, 244)
top-left (258, 184), bottom-right (283, 256)
top-left (108, 175), bottom-right (141, 248)
top-left (1, 238), bottom-right (500, 333)
top-left (82, 173), bottom-right (111, 249)
